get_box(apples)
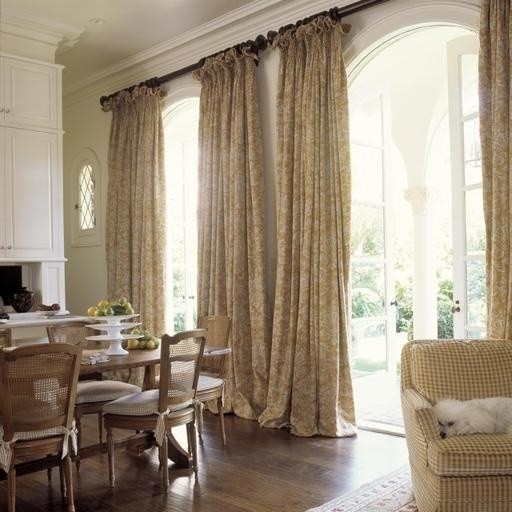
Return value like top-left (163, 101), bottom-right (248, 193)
top-left (128, 330), bottom-right (159, 348)
top-left (37, 304), bottom-right (59, 311)
top-left (87, 298), bottom-right (134, 316)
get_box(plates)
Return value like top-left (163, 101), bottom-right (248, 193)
top-left (36, 310), bottom-right (59, 317)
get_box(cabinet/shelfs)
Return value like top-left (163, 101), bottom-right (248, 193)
top-left (1, 54), bottom-right (63, 133)
top-left (1, 124), bottom-right (64, 260)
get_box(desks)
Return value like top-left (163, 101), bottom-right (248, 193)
top-left (0, 339), bottom-right (231, 470)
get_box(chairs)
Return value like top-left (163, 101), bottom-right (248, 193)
top-left (101, 328), bottom-right (207, 488)
top-left (0, 343), bottom-right (83, 512)
top-left (150, 316), bottom-right (232, 445)
top-left (46, 320), bottom-right (142, 452)
top-left (398, 337), bottom-right (512, 512)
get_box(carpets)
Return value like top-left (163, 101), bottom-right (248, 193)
top-left (300, 463), bottom-right (420, 511)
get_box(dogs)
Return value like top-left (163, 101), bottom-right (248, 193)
top-left (433, 397), bottom-right (512, 439)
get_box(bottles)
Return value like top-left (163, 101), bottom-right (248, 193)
top-left (11, 286), bottom-right (38, 313)
